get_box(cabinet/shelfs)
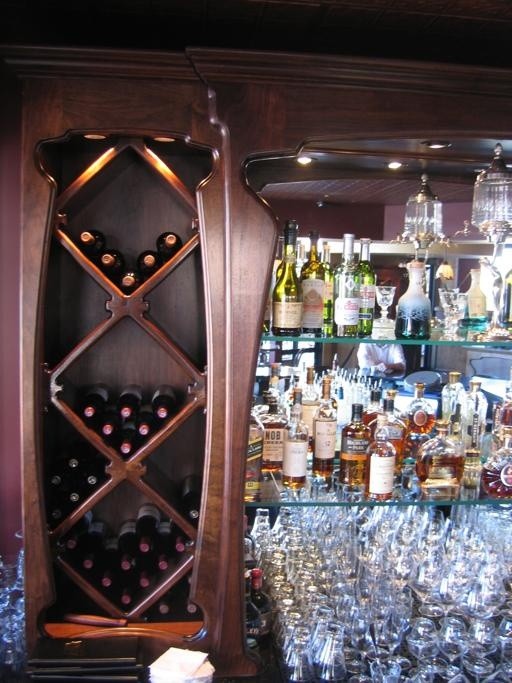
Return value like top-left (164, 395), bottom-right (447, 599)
top-left (185, 43), bottom-right (511, 680)
top-left (1, 38), bottom-right (258, 680)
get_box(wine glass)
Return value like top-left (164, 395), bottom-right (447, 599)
top-left (372, 284), bottom-right (398, 325)
top-left (438, 286), bottom-right (463, 332)
top-left (251, 507), bottom-right (512, 682)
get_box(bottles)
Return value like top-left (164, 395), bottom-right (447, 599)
top-left (237, 512), bottom-right (274, 656)
top-left (395, 261), bottom-right (432, 340)
top-left (78, 382), bottom-right (176, 455)
top-left (265, 218), bottom-right (378, 341)
top-left (460, 267), bottom-right (487, 333)
top-left (77, 229), bottom-right (183, 295)
top-left (43, 454), bottom-right (202, 623)
top-left (242, 362), bottom-right (511, 503)
